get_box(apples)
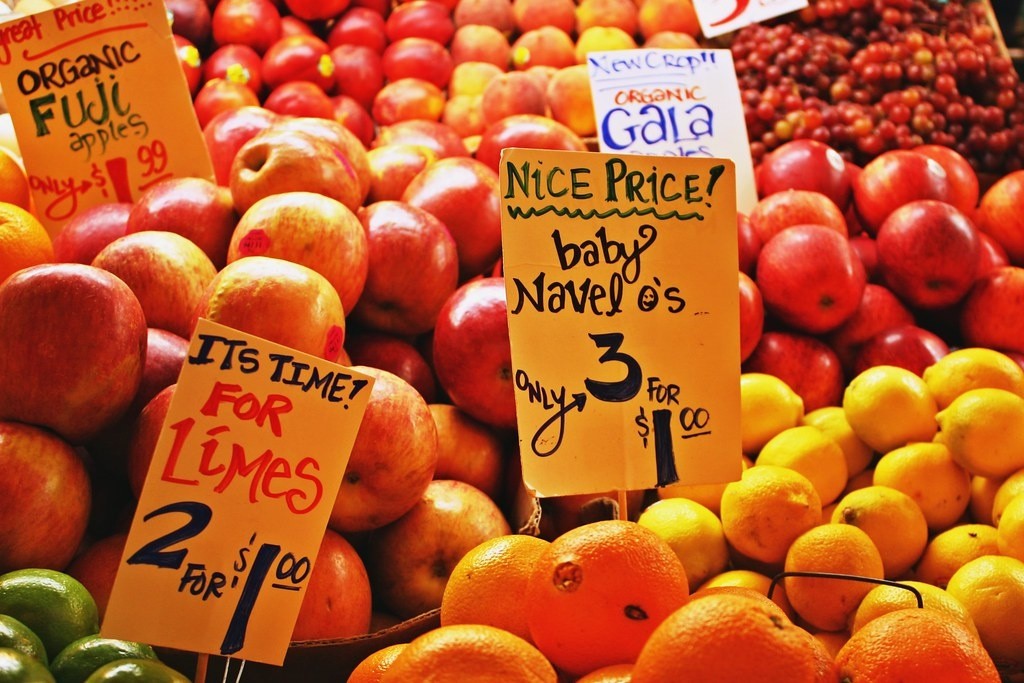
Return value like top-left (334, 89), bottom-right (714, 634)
top-left (737, 141), bottom-right (1024, 407)
top-left (0, 0), bottom-right (699, 642)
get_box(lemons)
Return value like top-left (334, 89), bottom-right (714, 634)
top-left (637, 346), bottom-right (1024, 683)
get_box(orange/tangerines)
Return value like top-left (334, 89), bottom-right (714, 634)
top-left (340, 521), bottom-right (1004, 681)
top-left (0, 569), bottom-right (198, 683)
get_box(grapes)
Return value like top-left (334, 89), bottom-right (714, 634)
top-left (730, 0), bottom-right (1024, 180)
